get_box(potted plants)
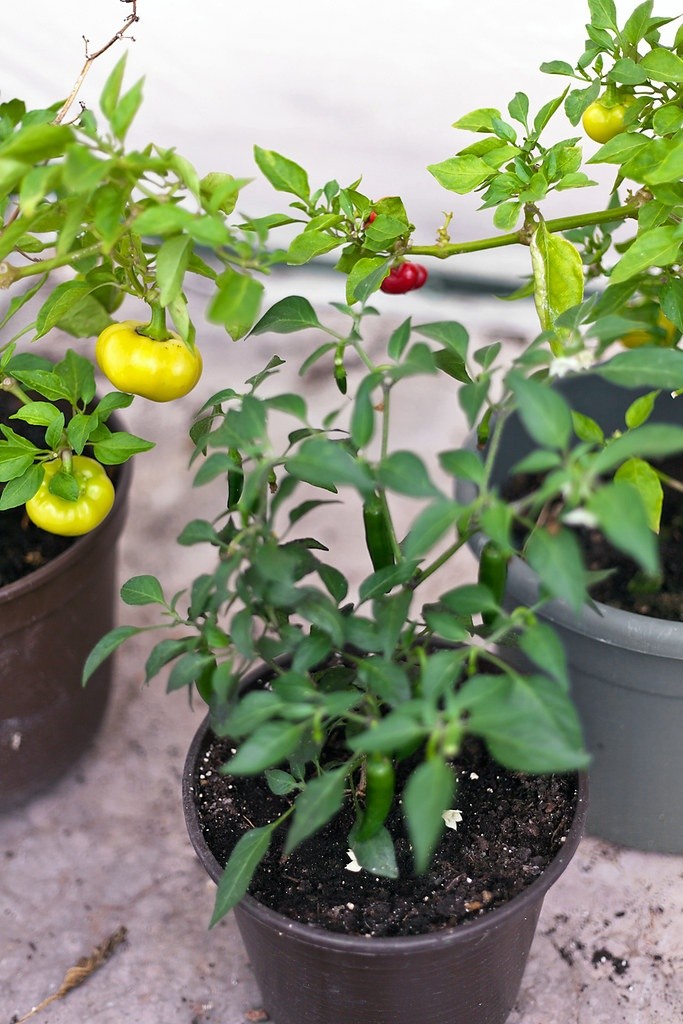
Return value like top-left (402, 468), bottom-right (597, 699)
top-left (0, 0), bottom-right (681, 1024)
